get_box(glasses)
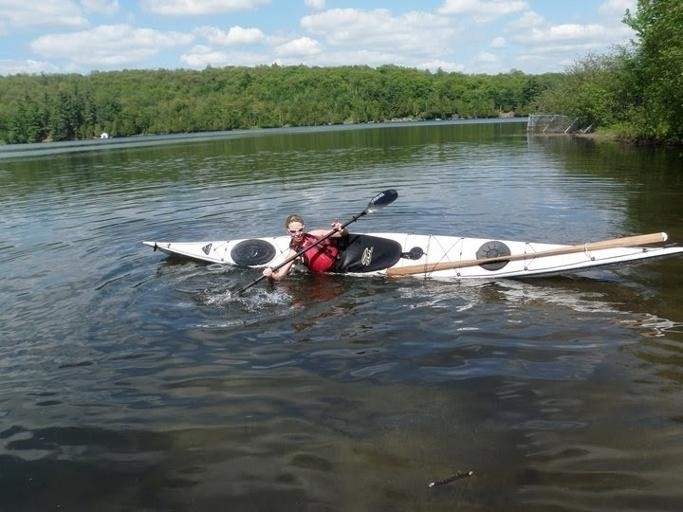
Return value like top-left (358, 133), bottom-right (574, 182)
top-left (290, 228), bottom-right (303, 233)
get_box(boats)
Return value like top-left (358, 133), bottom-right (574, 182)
top-left (142, 230), bottom-right (683, 281)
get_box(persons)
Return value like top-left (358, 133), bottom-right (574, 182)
top-left (258, 213), bottom-right (350, 282)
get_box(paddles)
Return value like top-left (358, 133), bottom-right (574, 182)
top-left (229, 190), bottom-right (398, 297)
top-left (386, 232), bottom-right (669, 277)
